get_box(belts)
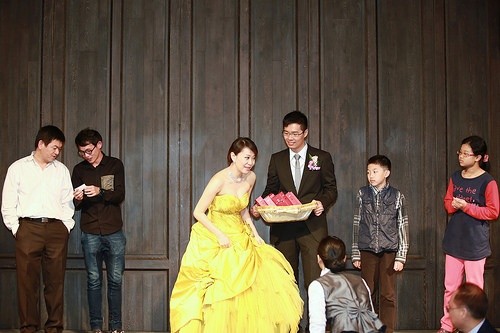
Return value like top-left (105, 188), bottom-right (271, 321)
top-left (23, 216), bottom-right (59, 223)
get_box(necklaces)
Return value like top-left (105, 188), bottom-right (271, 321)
top-left (228, 166), bottom-right (248, 183)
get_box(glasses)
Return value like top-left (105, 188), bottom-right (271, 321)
top-left (445, 305), bottom-right (461, 313)
top-left (282, 130), bottom-right (304, 138)
top-left (456, 151), bottom-right (477, 157)
top-left (77, 147), bottom-right (95, 156)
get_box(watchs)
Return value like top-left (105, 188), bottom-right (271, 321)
top-left (99, 188), bottom-right (105, 196)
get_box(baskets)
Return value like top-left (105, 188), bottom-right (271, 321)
top-left (257, 202), bottom-right (316, 223)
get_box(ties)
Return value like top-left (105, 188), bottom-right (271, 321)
top-left (293, 154), bottom-right (301, 194)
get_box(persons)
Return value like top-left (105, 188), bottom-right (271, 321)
top-left (307, 234), bottom-right (387, 333)
top-left (437, 133), bottom-right (500, 332)
top-left (192, 136), bottom-right (267, 333)
top-left (350, 154), bottom-right (409, 333)
top-left (249, 109), bottom-right (338, 332)
top-left (0, 125), bottom-right (76, 333)
top-left (447, 281), bottom-right (500, 332)
top-left (71, 127), bottom-right (125, 333)
